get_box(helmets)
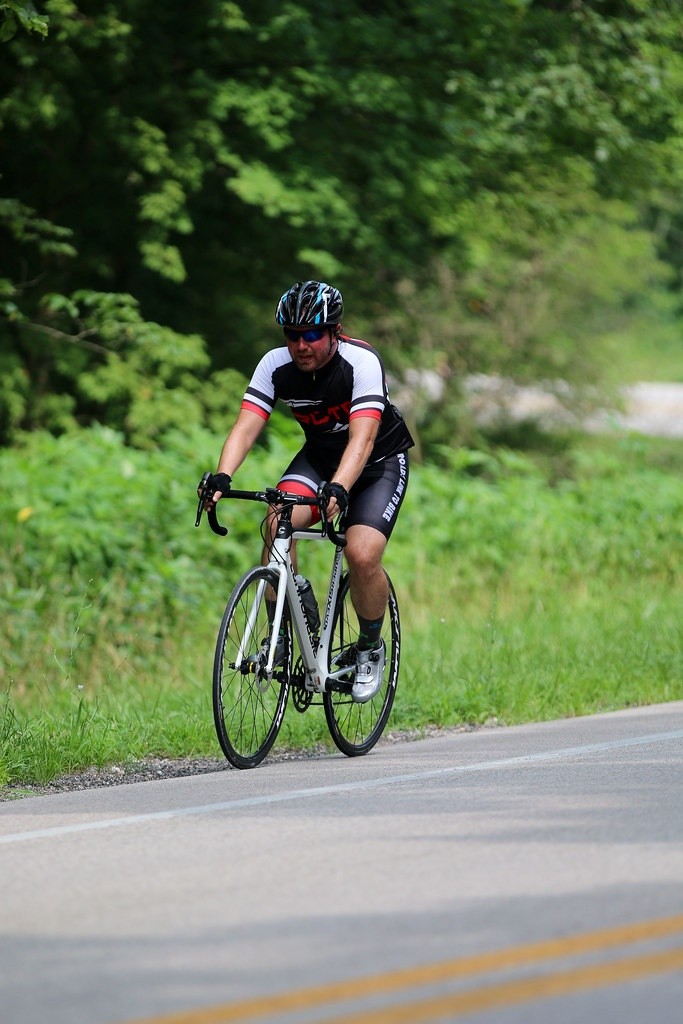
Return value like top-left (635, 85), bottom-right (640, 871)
top-left (275, 280), bottom-right (344, 326)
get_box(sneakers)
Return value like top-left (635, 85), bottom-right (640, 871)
top-left (352, 636), bottom-right (386, 703)
top-left (247, 635), bottom-right (284, 666)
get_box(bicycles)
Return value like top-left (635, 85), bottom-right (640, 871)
top-left (193, 471), bottom-right (402, 770)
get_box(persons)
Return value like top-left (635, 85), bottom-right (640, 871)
top-left (198, 280), bottom-right (416, 702)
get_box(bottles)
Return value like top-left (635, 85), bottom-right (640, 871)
top-left (295, 574), bottom-right (321, 631)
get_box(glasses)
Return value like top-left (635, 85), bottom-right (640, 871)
top-left (283, 326), bottom-right (327, 343)
